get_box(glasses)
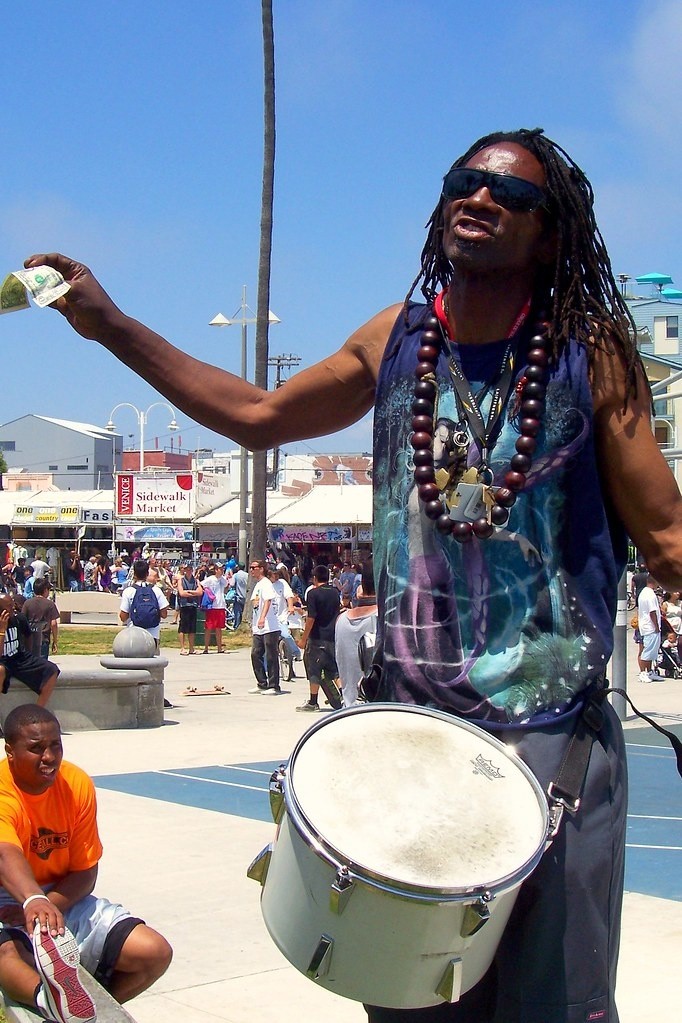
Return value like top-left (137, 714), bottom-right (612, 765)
top-left (442, 167), bottom-right (551, 214)
top-left (249, 566), bottom-right (257, 570)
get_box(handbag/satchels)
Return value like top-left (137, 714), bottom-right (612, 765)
top-left (630, 613), bottom-right (640, 631)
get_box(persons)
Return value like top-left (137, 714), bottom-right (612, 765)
top-left (0, 543), bottom-right (379, 709)
top-left (0, 703), bottom-right (173, 1023)
top-left (627, 563), bottom-right (682, 683)
top-left (25, 128), bottom-right (682, 1023)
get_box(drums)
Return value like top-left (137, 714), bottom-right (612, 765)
top-left (247, 702), bottom-right (551, 1010)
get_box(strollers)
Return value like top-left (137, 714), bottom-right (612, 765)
top-left (652, 616), bottom-right (682, 680)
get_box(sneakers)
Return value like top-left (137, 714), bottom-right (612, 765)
top-left (31, 918), bottom-right (97, 1023)
top-left (248, 687), bottom-right (265, 694)
top-left (295, 701), bottom-right (321, 713)
top-left (639, 671), bottom-right (661, 682)
top-left (261, 688), bottom-right (281, 695)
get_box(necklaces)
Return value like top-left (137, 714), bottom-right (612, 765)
top-left (410, 288), bottom-right (550, 544)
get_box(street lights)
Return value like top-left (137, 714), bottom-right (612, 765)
top-left (208, 284), bottom-right (282, 560)
top-left (104, 403), bottom-right (181, 472)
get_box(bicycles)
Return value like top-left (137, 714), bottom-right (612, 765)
top-left (254, 604), bottom-right (295, 682)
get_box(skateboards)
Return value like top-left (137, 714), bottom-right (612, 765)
top-left (179, 685), bottom-right (231, 696)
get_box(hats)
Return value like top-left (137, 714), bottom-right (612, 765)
top-left (214, 562), bottom-right (224, 568)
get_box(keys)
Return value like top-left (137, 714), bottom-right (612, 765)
top-left (483, 486), bottom-right (496, 526)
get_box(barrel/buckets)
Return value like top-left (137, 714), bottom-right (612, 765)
top-left (195, 608), bottom-right (217, 646)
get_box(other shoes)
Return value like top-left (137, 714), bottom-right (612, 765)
top-left (180, 651), bottom-right (189, 656)
top-left (218, 649), bottom-right (224, 653)
top-left (188, 649), bottom-right (197, 654)
top-left (203, 650), bottom-right (208, 654)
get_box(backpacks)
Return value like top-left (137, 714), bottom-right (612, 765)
top-left (131, 582), bottom-right (160, 628)
